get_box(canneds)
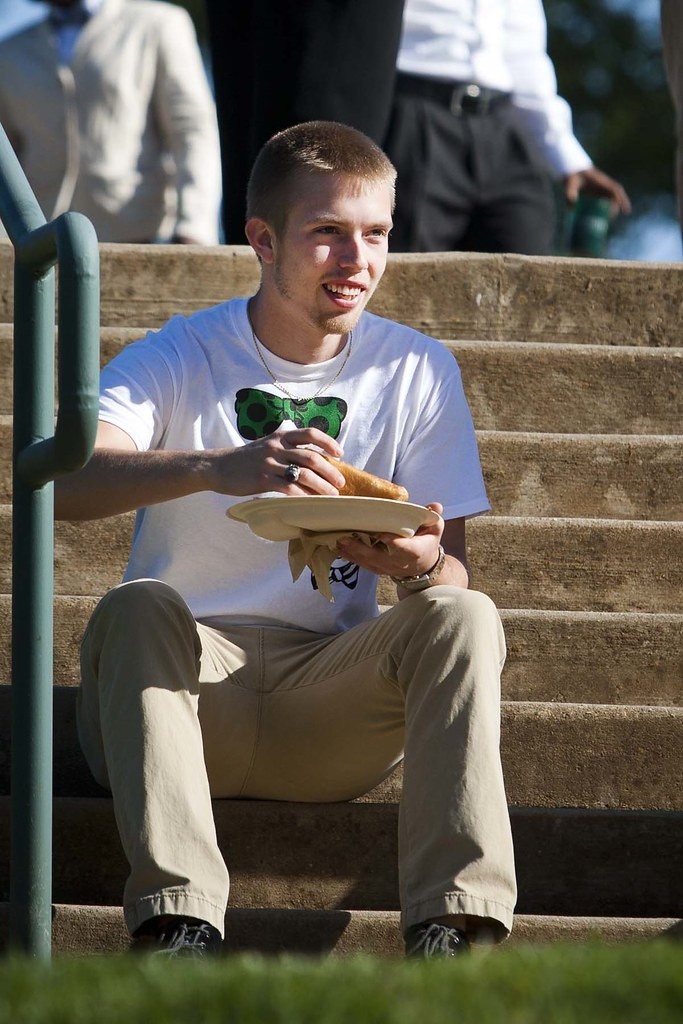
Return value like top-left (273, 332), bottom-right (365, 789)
top-left (570, 193), bottom-right (611, 258)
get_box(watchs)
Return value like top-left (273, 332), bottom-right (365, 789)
top-left (390, 544), bottom-right (446, 591)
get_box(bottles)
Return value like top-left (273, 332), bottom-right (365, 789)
top-left (573, 184), bottom-right (613, 257)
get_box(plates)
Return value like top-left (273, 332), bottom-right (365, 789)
top-left (226, 496), bottom-right (441, 541)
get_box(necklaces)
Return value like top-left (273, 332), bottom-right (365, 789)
top-left (249, 298), bottom-right (353, 399)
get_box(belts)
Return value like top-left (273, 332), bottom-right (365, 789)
top-left (395, 75), bottom-right (511, 118)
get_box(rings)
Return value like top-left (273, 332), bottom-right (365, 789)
top-left (285, 462), bottom-right (300, 482)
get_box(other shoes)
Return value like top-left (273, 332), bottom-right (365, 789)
top-left (154, 921), bottom-right (213, 977)
top-left (404, 921), bottom-right (471, 966)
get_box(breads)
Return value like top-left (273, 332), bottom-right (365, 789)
top-left (303, 447), bottom-right (408, 502)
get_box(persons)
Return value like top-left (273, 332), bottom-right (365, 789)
top-left (0, 0), bottom-right (631, 256)
top-left (53, 118), bottom-right (517, 966)
